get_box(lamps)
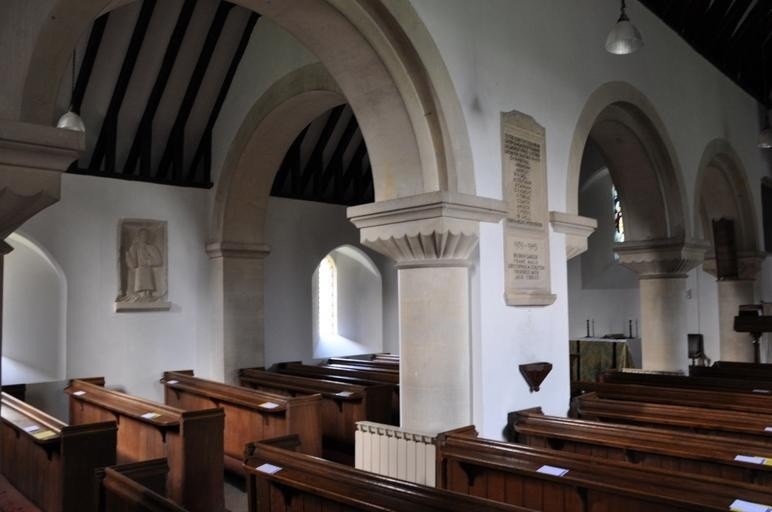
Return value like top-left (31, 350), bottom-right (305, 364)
top-left (758, 107), bottom-right (772, 149)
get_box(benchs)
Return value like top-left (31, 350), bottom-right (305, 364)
top-left (570, 393), bottom-right (772, 449)
top-left (605, 0), bottom-right (644, 54)
top-left (240, 434), bottom-right (539, 511)
top-left (57, 50), bottom-right (87, 133)
top-left (327, 355), bottom-right (399, 370)
top-left (241, 367), bottom-right (368, 440)
top-left (0, 384), bottom-right (120, 512)
top-left (159, 369), bottom-right (323, 491)
top-left (571, 381), bottom-right (772, 415)
top-left (277, 361), bottom-right (396, 424)
top-left (63, 377), bottom-right (225, 512)
top-left (572, 391), bottom-right (772, 437)
top-left (435, 426), bottom-right (772, 511)
top-left (506, 406), bottom-right (772, 486)
top-left (322, 359), bottom-right (398, 386)
